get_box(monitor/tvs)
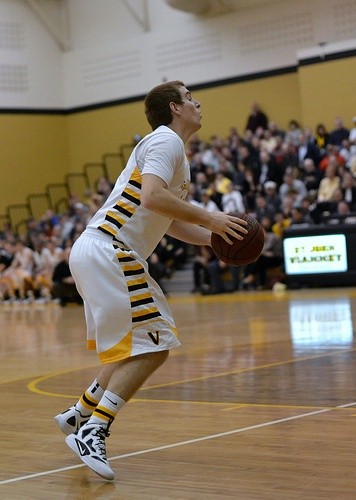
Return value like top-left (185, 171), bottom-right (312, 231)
top-left (282, 233), bottom-right (349, 275)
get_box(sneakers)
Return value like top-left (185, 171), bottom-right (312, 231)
top-left (54, 406), bottom-right (93, 435)
top-left (65, 422), bottom-right (114, 480)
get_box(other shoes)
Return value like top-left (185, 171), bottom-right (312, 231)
top-left (5, 297), bottom-right (58, 307)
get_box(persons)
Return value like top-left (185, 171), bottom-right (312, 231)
top-left (0, 101), bottom-right (356, 307)
top-left (52, 81), bottom-right (247, 480)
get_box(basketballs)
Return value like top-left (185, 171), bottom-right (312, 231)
top-left (210, 212), bottom-right (265, 267)
